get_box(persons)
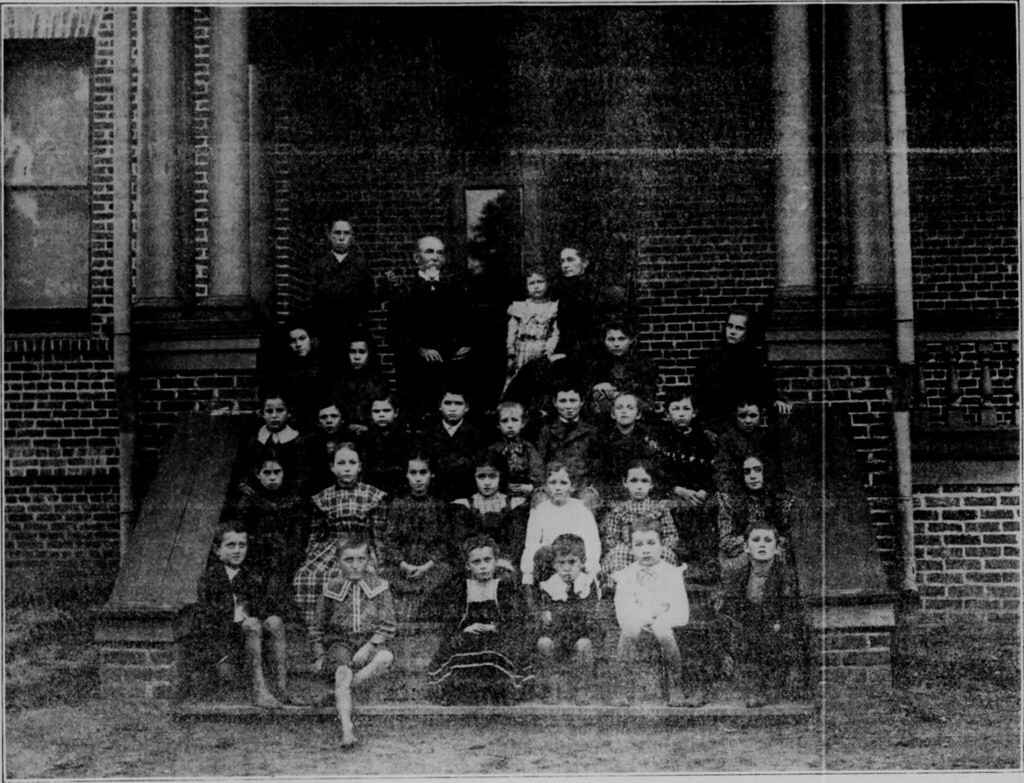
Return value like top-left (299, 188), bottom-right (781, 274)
top-left (229, 214), bottom-right (794, 639)
top-left (680, 519), bottom-right (805, 711)
top-left (523, 534), bottom-right (613, 706)
top-left (607, 515), bottom-right (693, 711)
top-left (422, 535), bottom-right (533, 708)
top-left (197, 520), bottom-right (308, 714)
top-left (305, 539), bottom-right (401, 748)
